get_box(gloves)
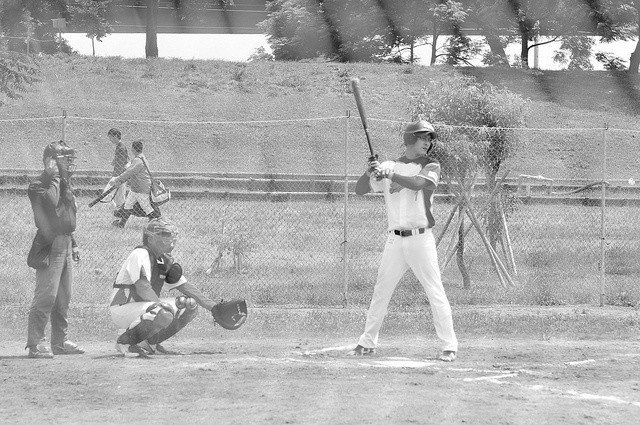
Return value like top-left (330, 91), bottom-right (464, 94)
top-left (372, 167), bottom-right (394, 182)
top-left (366, 161), bottom-right (381, 177)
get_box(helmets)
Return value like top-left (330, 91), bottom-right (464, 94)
top-left (42, 139), bottom-right (78, 173)
top-left (403, 120), bottom-right (438, 151)
top-left (143, 217), bottom-right (179, 236)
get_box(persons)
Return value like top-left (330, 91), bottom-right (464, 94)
top-left (109, 216), bottom-right (247, 358)
top-left (109, 141), bottom-right (171, 228)
top-left (103, 128), bottom-right (129, 209)
top-left (343, 119), bottom-right (458, 362)
top-left (26, 139), bottom-right (84, 359)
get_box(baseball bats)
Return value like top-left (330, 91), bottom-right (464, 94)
top-left (88, 185), bottom-right (116, 207)
top-left (351, 78), bottom-right (377, 161)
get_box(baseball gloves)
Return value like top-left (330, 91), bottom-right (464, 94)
top-left (211, 298), bottom-right (247, 330)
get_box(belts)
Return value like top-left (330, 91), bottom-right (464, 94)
top-left (394, 228), bottom-right (424, 236)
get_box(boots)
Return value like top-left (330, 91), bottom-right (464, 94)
top-left (112, 209), bottom-right (133, 228)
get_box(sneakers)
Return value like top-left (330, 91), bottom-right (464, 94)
top-left (28, 343), bottom-right (55, 359)
top-left (115, 341), bottom-right (144, 358)
top-left (141, 340), bottom-right (168, 355)
top-left (437, 351), bottom-right (456, 362)
top-left (345, 344), bottom-right (376, 358)
top-left (52, 340), bottom-right (86, 354)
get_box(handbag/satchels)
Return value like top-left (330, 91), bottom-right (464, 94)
top-left (27, 231), bottom-right (55, 269)
top-left (140, 156), bottom-right (171, 206)
